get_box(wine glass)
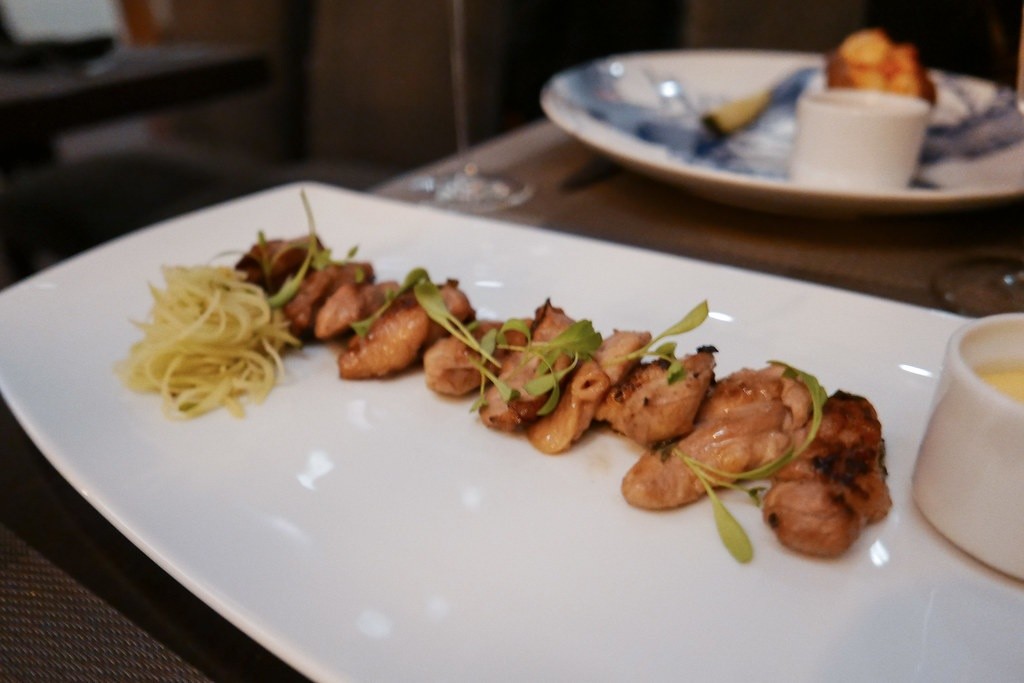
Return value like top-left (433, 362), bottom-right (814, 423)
top-left (406, 0), bottom-right (533, 215)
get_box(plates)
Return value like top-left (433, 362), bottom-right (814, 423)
top-left (0, 182), bottom-right (1022, 681)
top-left (541, 49), bottom-right (1024, 219)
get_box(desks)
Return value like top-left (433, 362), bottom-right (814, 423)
top-left (1, 41), bottom-right (276, 170)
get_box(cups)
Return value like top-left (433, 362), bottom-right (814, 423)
top-left (911, 314), bottom-right (1024, 581)
top-left (791, 91), bottom-right (929, 192)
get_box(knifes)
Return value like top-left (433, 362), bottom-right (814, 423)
top-left (700, 63), bottom-right (815, 139)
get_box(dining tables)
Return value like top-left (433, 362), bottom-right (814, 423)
top-left (366, 116), bottom-right (1024, 320)
